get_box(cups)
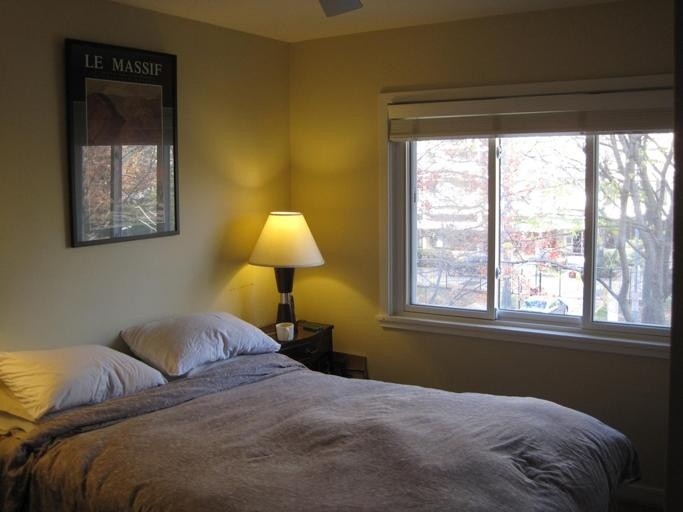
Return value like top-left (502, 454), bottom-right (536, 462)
top-left (275, 323), bottom-right (294, 342)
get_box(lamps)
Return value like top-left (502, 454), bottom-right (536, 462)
top-left (247, 211), bottom-right (325, 334)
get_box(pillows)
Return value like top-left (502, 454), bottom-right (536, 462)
top-left (0, 342), bottom-right (171, 424)
top-left (118, 310), bottom-right (283, 380)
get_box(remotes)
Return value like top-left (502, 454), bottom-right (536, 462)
top-left (303, 322), bottom-right (322, 332)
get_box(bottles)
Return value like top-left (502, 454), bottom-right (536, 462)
top-left (289, 295), bottom-right (296, 325)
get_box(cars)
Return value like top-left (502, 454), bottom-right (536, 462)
top-left (515, 295), bottom-right (569, 316)
top-left (415, 246), bottom-right (635, 283)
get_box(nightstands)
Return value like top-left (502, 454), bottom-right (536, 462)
top-left (260, 320), bottom-right (335, 375)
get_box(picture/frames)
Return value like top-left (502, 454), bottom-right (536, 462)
top-left (63, 36), bottom-right (181, 249)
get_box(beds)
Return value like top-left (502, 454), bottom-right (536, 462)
top-left (0, 359), bottom-right (643, 512)
